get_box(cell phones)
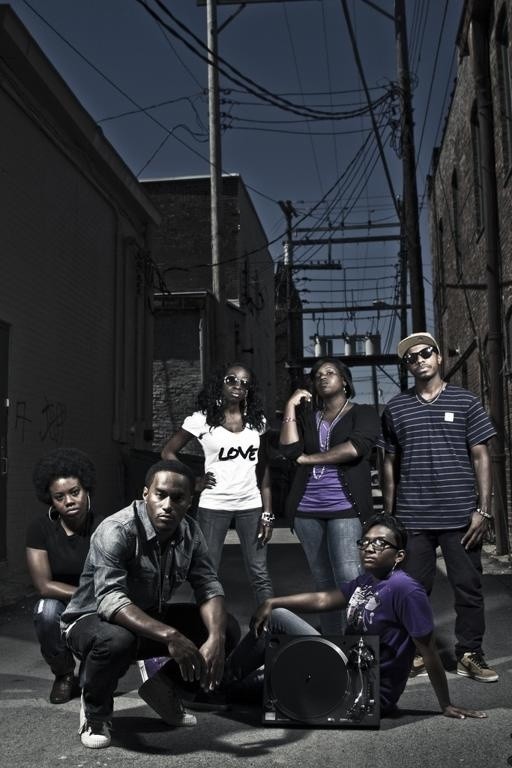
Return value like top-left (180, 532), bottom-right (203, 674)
top-left (257, 624), bottom-right (268, 640)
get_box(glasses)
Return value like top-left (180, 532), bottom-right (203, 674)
top-left (356, 538), bottom-right (398, 552)
top-left (223, 374), bottom-right (250, 388)
top-left (405, 346), bottom-right (434, 362)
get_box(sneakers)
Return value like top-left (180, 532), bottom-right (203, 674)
top-left (49, 674), bottom-right (79, 703)
top-left (181, 681), bottom-right (235, 711)
top-left (77, 696), bottom-right (113, 749)
top-left (456, 653), bottom-right (499, 683)
top-left (137, 677), bottom-right (197, 728)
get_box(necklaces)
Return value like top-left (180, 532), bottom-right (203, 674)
top-left (311, 398), bottom-right (348, 481)
top-left (415, 380), bottom-right (444, 406)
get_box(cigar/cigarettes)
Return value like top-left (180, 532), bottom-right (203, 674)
top-left (192, 663), bottom-right (195, 672)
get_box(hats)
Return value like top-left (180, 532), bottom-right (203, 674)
top-left (397, 332), bottom-right (441, 359)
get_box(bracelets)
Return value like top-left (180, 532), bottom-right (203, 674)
top-left (476, 508), bottom-right (494, 521)
top-left (281, 416), bottom-right (297, 425)
top-left (260, 512), bottom-right (276, 527)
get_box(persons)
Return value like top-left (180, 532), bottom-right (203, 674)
top-left (278, 359), bottom-right (382, 640)
top-left (137, 514), bottom-right (488, 720)
top-left (60, 459), bottom-right (241, 749)
top-left (162, 363), bottom-right (274, 615)
top-left (25, 462), bottom-right (112, 704)
top-left (377, 333), bottom-right (498, 683)
